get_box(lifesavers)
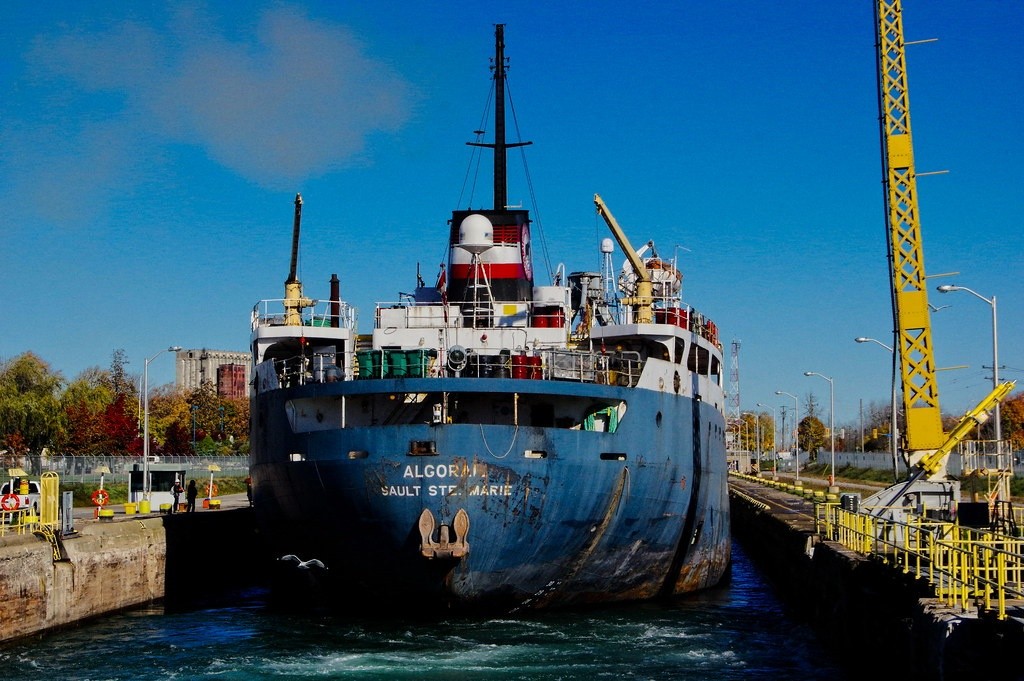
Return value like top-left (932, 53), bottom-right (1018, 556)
top-left (92, 490), bottom-right (109, 506)
top-left (205, 484), bottom-right (217, 497)
top-left (1, 494), bottom-right (20, 511)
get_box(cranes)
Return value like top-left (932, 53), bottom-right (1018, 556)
top-left (859, 3), bottom-right (1017, 538)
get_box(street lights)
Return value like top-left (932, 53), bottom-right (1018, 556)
top-left (776, 391), bottom-right (801, 485)
top-left (726, 412), bottom-right (761, 478)
top-left (804, 372), bottom-right (839, 494)
top-left (937, 284), bottom-right (1003, 470)
top-left (138, 346), bottom-right (182, 514)
top-left (757, 403), bottom-right (779, 481)
top-left (854, 337), bottom-right (900, 483)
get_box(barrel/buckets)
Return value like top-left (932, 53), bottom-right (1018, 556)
top-left (314, 369), bottom-right (326, 384)
top-left (327, 369), bottom-right (337, 382)
top-left (547, 305), bottom-right (564, 328)
top-left (533, 307), bottom-right (549, 327)
top-left (656, 307), bottom-right (692, 331)
top-left (447, 354), bottom-right (542, 380)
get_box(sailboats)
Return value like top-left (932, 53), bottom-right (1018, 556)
top-left (242, 24), bottom-right (732, 628)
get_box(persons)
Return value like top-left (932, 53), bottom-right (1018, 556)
top-left (170, 479), bottom-right (198, 512)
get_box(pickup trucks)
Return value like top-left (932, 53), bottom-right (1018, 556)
top-left (0, 481), bottom-right (42, 516)
